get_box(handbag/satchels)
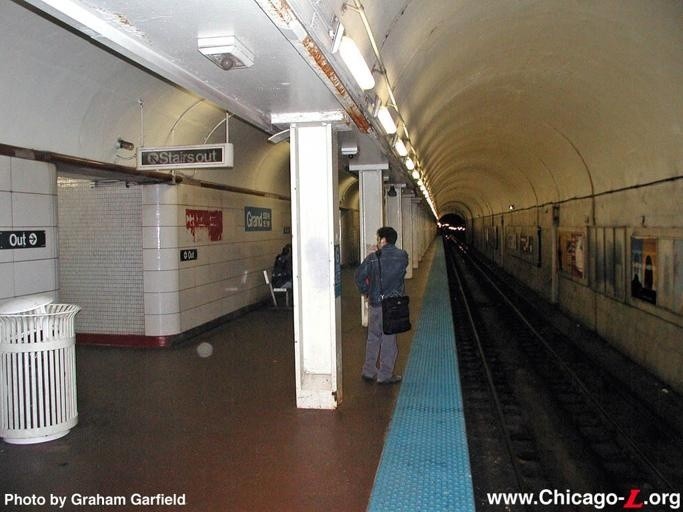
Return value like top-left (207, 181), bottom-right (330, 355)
top-left (378, 294), bottom-right (414, 337)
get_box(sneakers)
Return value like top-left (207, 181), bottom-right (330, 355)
top-left (361, 371), bottom-right (377, 383)
top-left (376, 373), bottom-right (402, 385)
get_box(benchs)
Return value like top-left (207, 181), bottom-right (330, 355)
top-left (262, 268), bottom-right (292, 311)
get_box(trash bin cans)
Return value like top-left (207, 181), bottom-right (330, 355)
top-left (0, 295), bottom-right (82, 445)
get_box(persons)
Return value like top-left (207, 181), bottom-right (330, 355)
top-left (352, 226), bottom-right (410, 386)
top-left (282, 247), bottom-right (291, 268)
top-left (271, 253), bottom-right (291, 290)
